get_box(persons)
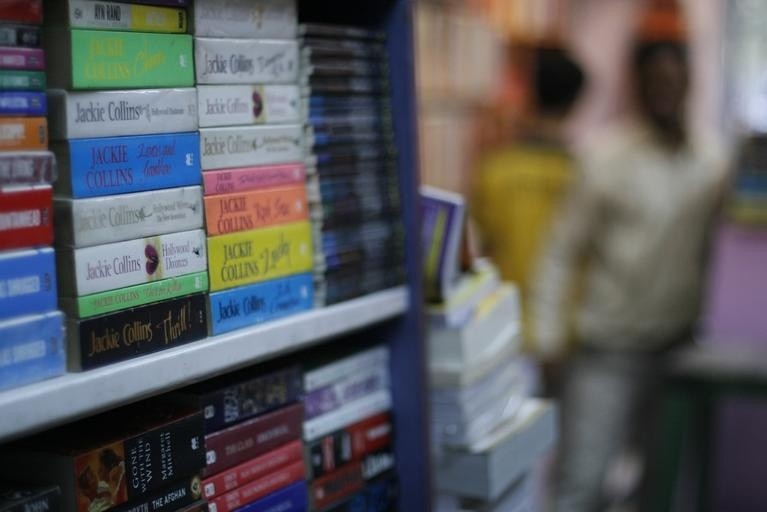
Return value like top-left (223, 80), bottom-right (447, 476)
top-left (469, 27), bottom-right (766, 512)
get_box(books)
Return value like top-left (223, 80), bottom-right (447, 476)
top-left (1, 344), bottom-right (401, 512)
top-left (416, 178), bottom-right (558, 511)
top-left (1, 1), bottom-right (411, 393)
